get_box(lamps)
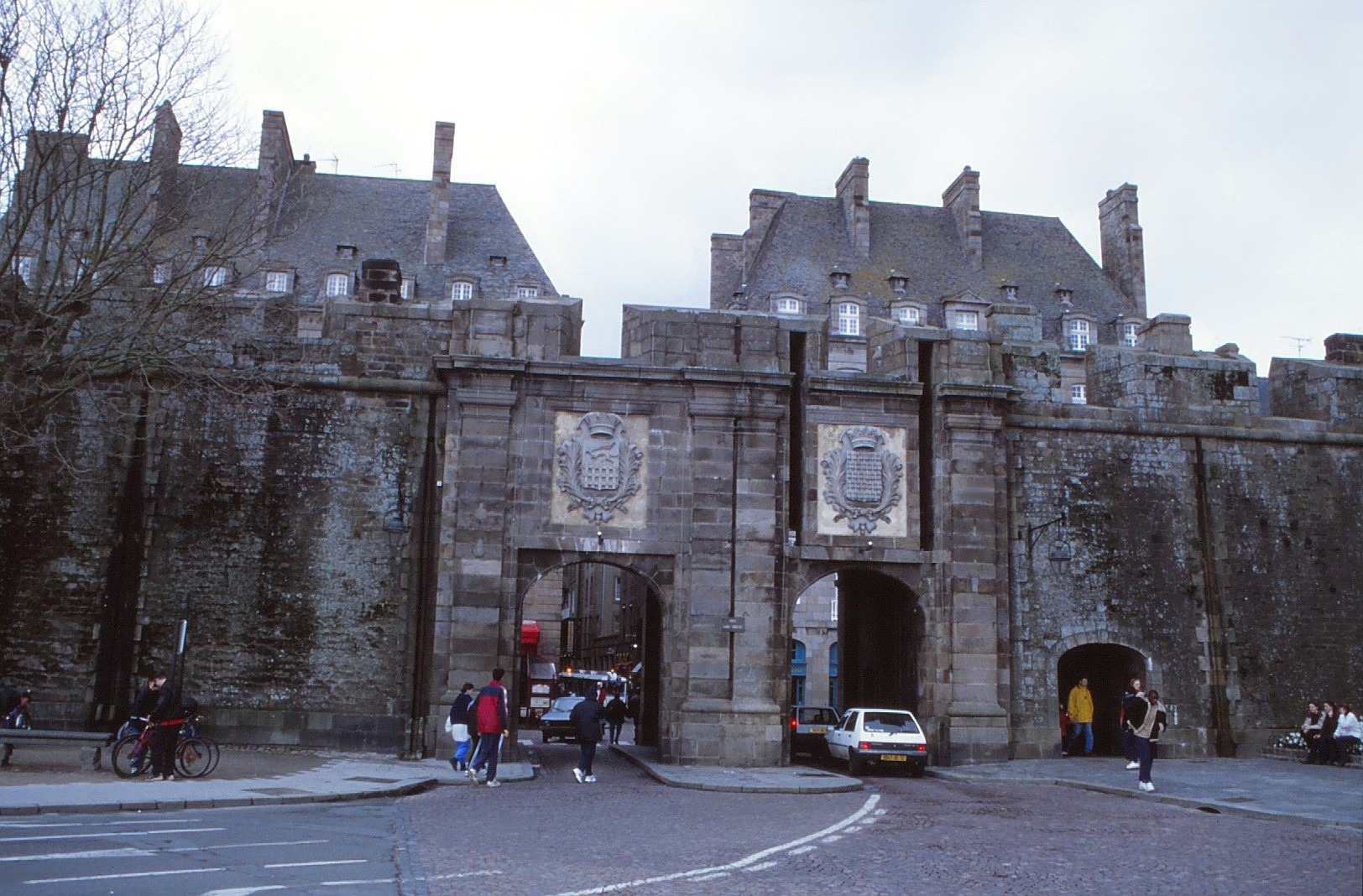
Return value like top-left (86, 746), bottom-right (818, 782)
top-left (597, 531), bottom-right (603, 545)
top-left (381, 481), bottom-right (410, 553)
top-left (1027, 509), bottom-right (1071, 575)
top-left (858, 541), bottom-right (874, 553)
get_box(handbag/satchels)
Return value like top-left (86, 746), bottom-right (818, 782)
top-left (445, 715), bottom-right (452, 733)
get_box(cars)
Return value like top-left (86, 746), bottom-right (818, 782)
top-left (539, 697), bottom-right (606, 742)
top-left (823, 708), bottom-right (927, 778)
top-left (790, 704), bottom-right (842, 749)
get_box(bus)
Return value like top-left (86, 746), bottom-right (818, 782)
top-left (558, 668), bottom-right (629, 713)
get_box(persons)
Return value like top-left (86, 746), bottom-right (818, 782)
top-left (127, 668), bottom-right (199, 782)
top-left (449, 668), bottom-right (508, 788)
top-left (569, 689), bottom-right (606, 784)
top-left (1299, 700), bottom-right (1361, 768)
top-left (606, 687), bottom-right (640, 745)
top-left (1059, 678), bottom-right (1093, 758)
top-left (1120, 679), bottom-right (1169, 792)
top-left (0, 692), bottom-right (34, 767)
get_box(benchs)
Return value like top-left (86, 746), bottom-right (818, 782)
top-left (0, 728), bottom-right (113, 770)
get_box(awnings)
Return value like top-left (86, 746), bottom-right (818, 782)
top-left (631, 661), bottom-right (641, 675)
top-left (521, 620), bottom-right (540, 645)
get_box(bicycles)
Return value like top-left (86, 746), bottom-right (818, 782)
top-left (111, 712), bottom-right (220, 778)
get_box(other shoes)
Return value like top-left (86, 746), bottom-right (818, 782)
top-left (1139, 781), bottom-right (1154, 792)
top-left (464, 770), bottom-right (469, 776)
top-left (145, 772), bottom-right (163, 781)
top-left (573, 768), bottom-right (583, 784)
top-left (1126, 761), bottom-right (1140, 769)
top-left (449, 758), bottom-right (457, 771)
top-left (164, 774), bottom-right (175, 781)
top-left (480, 769), bottom-right (487, 778)
top-left (486, 778), bottom-right (501, 787)
top-left (470, 769), bottom-right (479, 787)
top-left (459, 767), bottom-right (467, 772)
top-left (1301, 753), bottom-right (1351, 767)
top-left (585, 775), bottom-right (597, 783)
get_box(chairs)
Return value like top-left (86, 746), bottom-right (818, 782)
top-left (812, 715), bottom-right (825, 725)
